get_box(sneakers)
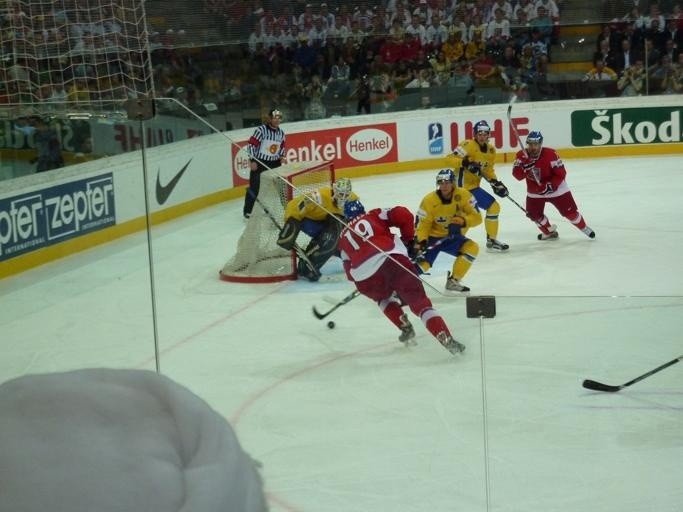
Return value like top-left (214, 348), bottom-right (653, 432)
top-left (436, 332), bottom-right (466, 354)
top-left (583, 226), bottom-right (595, 238)
top-left (397, 314), bottom-right (413, 341)
top-left (486, 234), bottom-right (508, 250)
top-left (445, 271), bottom-right (469, 291)
top-left (537, 230), bottom-right (558, 240)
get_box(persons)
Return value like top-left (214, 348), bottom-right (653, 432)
top-left (276, 177), bottom-right (366, 282)
top-left (243, 110), bottom-right (289, 219)
top-left (338, 199), bottom-right (465, 354)
top-left (0, 0), bottom-right (683, 173)
top-left (445, 119), bottom-right (509, 251)
top-left (393, 170), bottom-right (483, 308)
top-left (512, 130), bottom-right (596, 240)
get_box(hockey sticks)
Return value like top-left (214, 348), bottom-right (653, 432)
top-left (582, 356), bottom-right (682, 392)
top-left (476, 171), bottom-right (557, 233)
top-left (506, 95), bottom-right (541, 185)
top-left (245, 187), bottom-right (347, 282)
top-left (313, 236), bottom-right (455, 320)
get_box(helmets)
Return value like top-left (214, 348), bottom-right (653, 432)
top-left (474, 120), bottom-right (490, 135)
top-left (344, 201), bottom-right (364, 220)
top-left (269, 109), bottom-right (283, 121)
top-left (332, 177), bottom-right (350, 205)
top-left (526, 130), bottom-right (543, 145)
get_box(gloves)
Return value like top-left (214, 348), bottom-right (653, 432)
top-left (462, 156), bottom-right (480, 175)
top-left (537, 181), bottom-right (552, 196)
top-left (406, 240), bottom-right (417, 258)
top-left (520, 158), bottom-right (535, 172)
top-left (492, 179), bottom-right (508, 196)
top-left (448, 223), bottom-right (462, 241)
